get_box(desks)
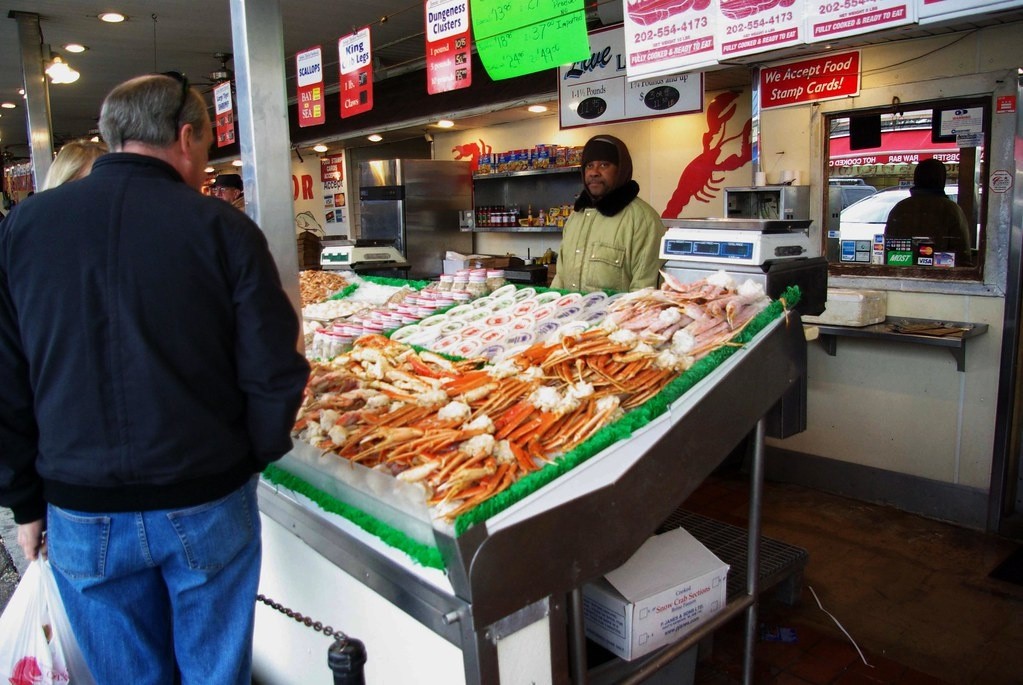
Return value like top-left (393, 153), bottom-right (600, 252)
top-left (322, 263), bottom-right (413, 280)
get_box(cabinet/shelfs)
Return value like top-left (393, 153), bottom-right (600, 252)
top-left (472, 165), bottom-right (585, 233)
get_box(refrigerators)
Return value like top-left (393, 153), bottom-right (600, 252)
top-left (357, 159), bottom-right (474, 280)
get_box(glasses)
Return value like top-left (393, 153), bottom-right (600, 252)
top-left (149, 71), bottom-right (191, 141)
top-left (212, 187), bottom-right (231, 193)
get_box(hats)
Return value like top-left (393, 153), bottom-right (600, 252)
top-left (209, 174), bottom-right (243, 192)
top-left (582, 138), bottom-right (619, 166)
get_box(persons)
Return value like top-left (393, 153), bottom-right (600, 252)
top-left (549, 134), bottom-right (665, 291)
top-left (42, 141), bottom-right (108, 190)
top-left (0, 69), bottom-right (311, 685)
top-left (208, 174), bottom-right (245, 212)
top-left (884, 158), bottom-right (972, 266)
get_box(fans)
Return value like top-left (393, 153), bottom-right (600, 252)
top-left (186, 53), bottom-right (235, 94)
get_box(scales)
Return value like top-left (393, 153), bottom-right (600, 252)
top-left (655, 214), bottom-right (830, 316)
top-left (320, 238), bottom-right (412, 272)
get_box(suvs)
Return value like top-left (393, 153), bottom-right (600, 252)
top-left (827, 178), bottom-right (878, 209)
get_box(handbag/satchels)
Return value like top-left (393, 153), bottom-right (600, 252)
top-left (0, 551), bottom-right (97, 685)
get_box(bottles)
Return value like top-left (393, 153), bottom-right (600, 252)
top-left (527, 204), bottom-right (534, 227)
top-left (539, 210), bottom-right (544, 227)
top-left (475, 203), bottom-right (521, 227)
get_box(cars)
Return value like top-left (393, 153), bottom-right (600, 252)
top-left (840, 184), bottom-right (980, 250)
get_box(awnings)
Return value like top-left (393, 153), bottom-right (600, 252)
top-left (827, 126), bottom-right (1023, 171)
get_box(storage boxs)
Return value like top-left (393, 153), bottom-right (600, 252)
top-left (801, 288), bottom-right (888, 327)
top-left (581, 527), bottom-right (731, 661)
top-left (589, 645), bottom-right (697, 685)
